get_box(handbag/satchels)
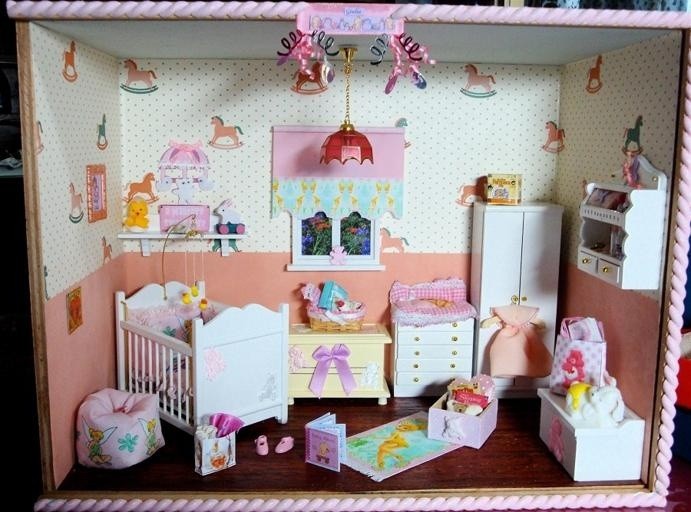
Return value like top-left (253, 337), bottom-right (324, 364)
top-left (194, 431), bottom-right (236, 475)
top-left (549, 335), bottom-right (606, 396)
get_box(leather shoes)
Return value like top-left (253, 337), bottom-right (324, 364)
top-left (275, 437), bottom-right (294, 453)
top-left (255, 436), bottom-right (268, 455)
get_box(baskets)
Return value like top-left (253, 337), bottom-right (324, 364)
top-left (306, 302), bottom-right (367, 331)
